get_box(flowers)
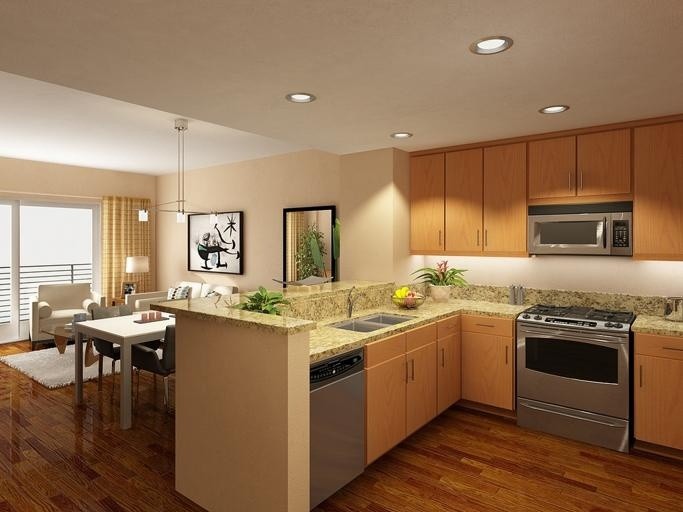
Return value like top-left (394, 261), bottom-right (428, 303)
top-left (296, 220), bottom-right (326, 281)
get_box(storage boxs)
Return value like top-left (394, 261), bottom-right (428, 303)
top-left (461, 311), bottom-right (515, 413)
top-left (633, 113), bottom-right (683, 260)
top-left (437, 314), bottom-right (461, 420)
top-left (526, 122), bottom-right (633, 204)
top-left (635, 331), bottom-right (683, 462)
top-left (365, 322), bottom-right (438, 465)
top-left (446, 136), bottom-right (528, 256)
top-left (409, 147), bottom-right (446, 255)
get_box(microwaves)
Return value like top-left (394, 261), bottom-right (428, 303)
top-left (525, 212), bottom-right (635, 257)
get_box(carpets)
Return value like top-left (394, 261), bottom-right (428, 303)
top-left (0, 339), bottom-right (145, 390)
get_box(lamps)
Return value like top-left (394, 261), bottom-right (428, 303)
top-left (117, 199), bottom-right (186, 230)
top-left (126, 255), bottom-right (149, 293)
top-left (161, 120), bottom-right (219, 226)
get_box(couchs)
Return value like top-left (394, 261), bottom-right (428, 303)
top-left (30, 282), bottom-right (99, 352)
top-left (125, 281), bottom-right (238, 312)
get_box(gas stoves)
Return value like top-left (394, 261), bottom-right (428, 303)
top-left (514, 304), bottom-right (636, 453)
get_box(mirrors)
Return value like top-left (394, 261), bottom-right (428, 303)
top-left (282, 205), bottom-right (336, 288)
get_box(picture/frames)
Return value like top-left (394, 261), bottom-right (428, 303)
top-left (187, 210), bottom-right (243, 274)
top-left (119, 280), bottom-right (140, 299)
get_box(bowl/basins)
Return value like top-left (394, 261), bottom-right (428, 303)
top-left (390, 295), bottom-right (425, 308)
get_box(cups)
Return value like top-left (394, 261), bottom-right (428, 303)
top-left (149, 312), bottom-right (154, 321)
top-left (142, 314), bottom-right (147, 322)
top-left (156, 311), bottom-right (161, 320)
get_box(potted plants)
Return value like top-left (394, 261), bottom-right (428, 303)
top-left (409, 260), bottom-right (468, 305)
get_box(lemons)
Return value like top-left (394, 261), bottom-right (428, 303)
top-left (415, 299), bottom-right (424, 306)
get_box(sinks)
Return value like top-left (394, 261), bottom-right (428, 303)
top-left (363, 312), bottom-right (419, 325)
top-left (323, 318), bottom-right (389, 334)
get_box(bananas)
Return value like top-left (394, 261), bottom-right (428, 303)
top-left (392, 287), bottom-right (409, 306)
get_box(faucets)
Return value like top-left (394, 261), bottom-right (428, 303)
top-left (347, 286), bottom-right (366, 319)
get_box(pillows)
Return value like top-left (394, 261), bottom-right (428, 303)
top-left (202, 288), bottom-right (223, 298)
top-left (166, 285), bottom-right (190, 301)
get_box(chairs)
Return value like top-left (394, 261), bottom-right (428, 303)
top-left (91, 305), bottom-right (134, 394)
top-left (131, 324), bottom-right (176, 415)
